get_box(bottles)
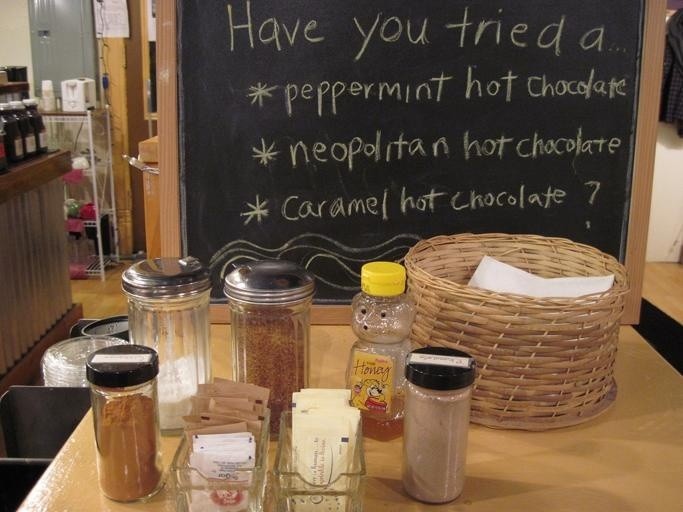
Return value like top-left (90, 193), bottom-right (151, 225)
top-left (117, 253), bottom-right (213, 438)
top-left (87, 347), bottom-right (165, 503)
top-left (345, 260), bottom-right (413, 443)
top-left (225, 261), bottom-right (316, 439)
top-left (404, 345), bottom-right (474, 505)
top-left (0, 98), bottom-right (49, 175)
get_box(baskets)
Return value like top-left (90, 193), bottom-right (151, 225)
top-left (403, 231), bottom-right (632, 432)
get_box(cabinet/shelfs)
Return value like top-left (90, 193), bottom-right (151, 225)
top-left (38, 107), bottom-right (121, 281)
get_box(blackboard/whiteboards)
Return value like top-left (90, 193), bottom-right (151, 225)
top-left (155, 0), bottom-right (667, 325)
